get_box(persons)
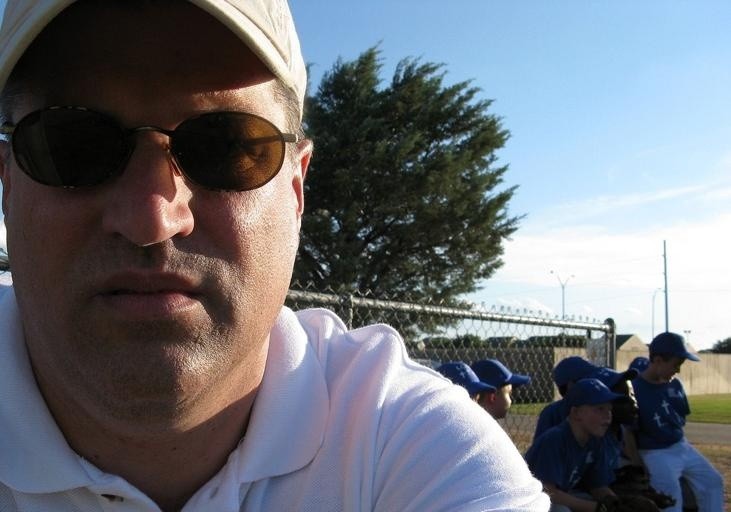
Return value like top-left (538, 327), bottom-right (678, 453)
top-left (435, 360), bottom-right (498, 404)
top-left (470, 357), bottom-right (533, 419)
top-left (524, 332), bottom-right (725, 511)
top-left (1, 1), bottom-right (554, 512)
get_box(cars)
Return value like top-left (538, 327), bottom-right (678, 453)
top-left (411, 358), bottom-right (441, 372)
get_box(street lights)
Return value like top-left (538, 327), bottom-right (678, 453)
top-left (681, 329), bottom-right (693, 346)
top-left (548, 268), bottom-right (578, 318)
top-left (651, 285), bottom-right (662, 346)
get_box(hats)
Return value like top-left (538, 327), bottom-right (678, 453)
top-left (433, 363), bottom-right (498, 393)
top-left (551, 332), bottom-right (700, 406)
top-left (0, 0), bottom-right (306, 125)
top-left (475, 360), bottom-right (530, 387)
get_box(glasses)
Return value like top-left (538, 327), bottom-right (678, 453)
top-left (0, 108), bottom-right (298, 193)
